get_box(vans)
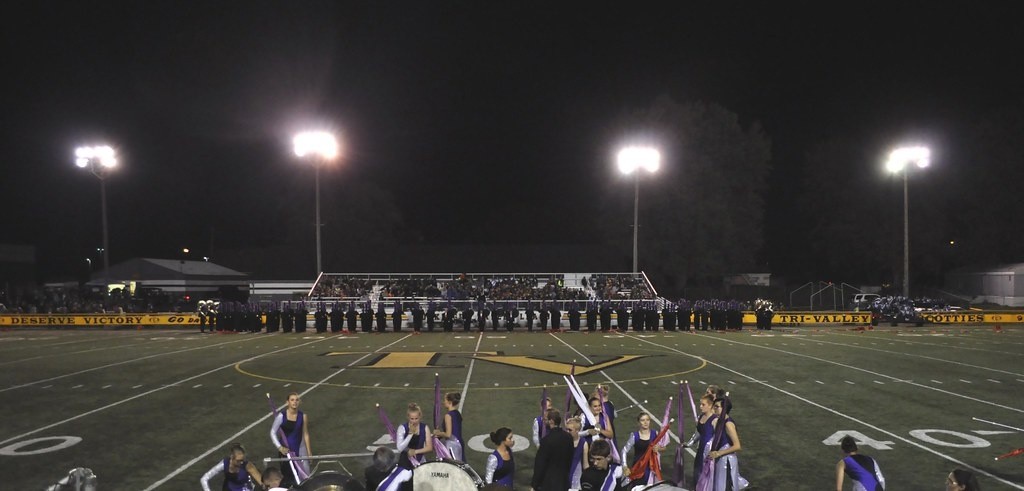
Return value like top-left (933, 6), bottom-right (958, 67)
top-left (853, 293), bottom-right (880, 303)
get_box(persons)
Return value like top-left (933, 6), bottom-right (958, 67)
top-left (836, 437), bottom-right (889, 491)
top-left (945, 469), bottom-right (980, 491)
top-left (201, 272), bottom-right (773, 332)
top-left (200, 383), bottom-right (749, 491)
top-left (0, 283), bottom-right (165, 314)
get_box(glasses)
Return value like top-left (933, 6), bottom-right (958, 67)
top-left (713, 405), bottom-right (721, 409)
top-left (589, 458), bottom-right (607, 463)
top-left (947, 478), bottom-right (958, 486)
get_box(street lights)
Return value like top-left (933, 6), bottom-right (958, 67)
top-left (293, 130), bottom-right (339, 278)
top-left (885, 147), bottom-right (930, 295)
top-left (616, 145), bottom-right (661, 277)
top-left (77, 147), bottom-right (122, 270)
